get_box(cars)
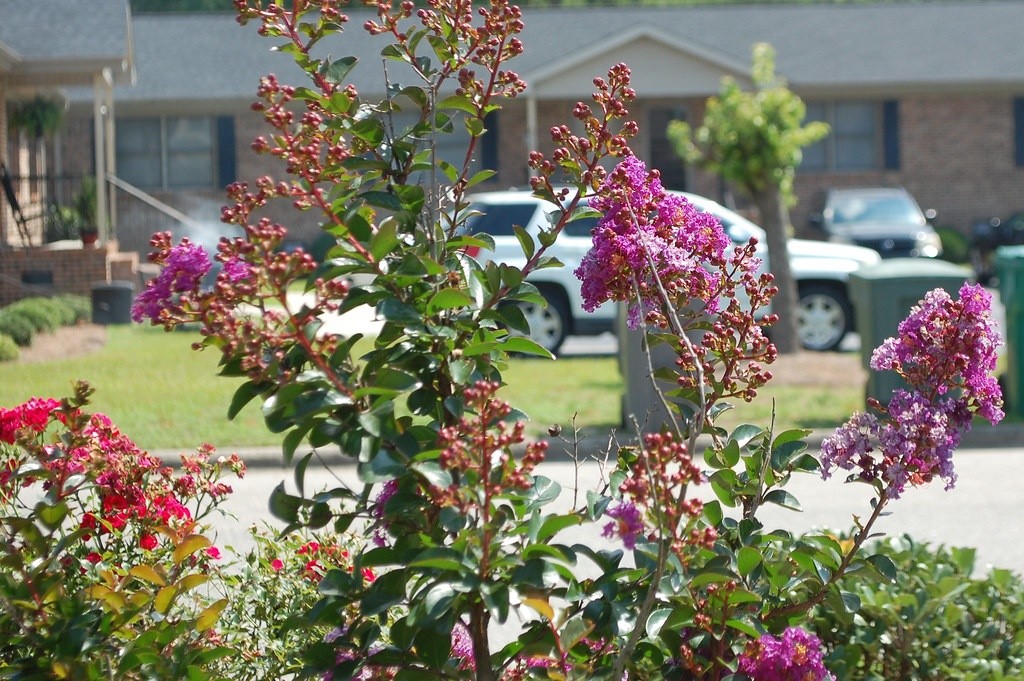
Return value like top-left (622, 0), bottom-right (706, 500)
top-left (967, 209), bottom-right (1024, 278)
top-left (810, 177), bottom-right (943, 262)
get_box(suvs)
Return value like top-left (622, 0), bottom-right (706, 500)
top-left (445, 184), bottom-right (883, 358)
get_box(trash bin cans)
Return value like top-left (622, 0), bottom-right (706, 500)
top-left (848, 257), bottom-right (966, 424)
top-left (92, 283), bottom-right (132, 321)
top-left (990, 244), bottom-right (1024, 421)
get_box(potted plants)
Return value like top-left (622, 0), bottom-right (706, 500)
top-left (8, 97), bottom-right (61, 143)
top-left (74, 171), bottom-right (97, 246)
